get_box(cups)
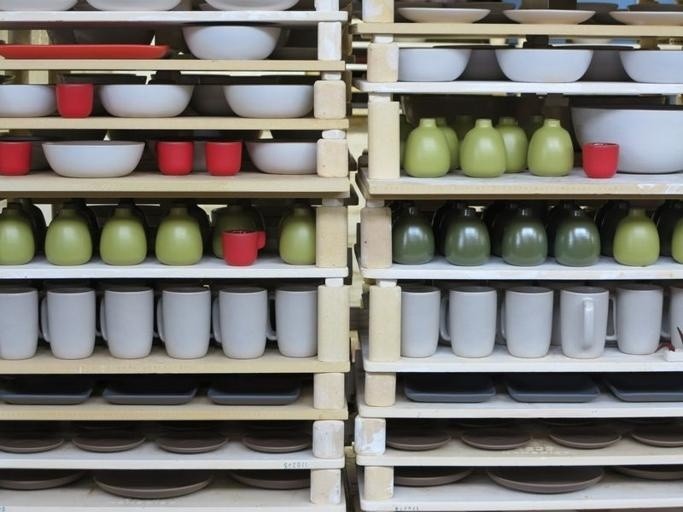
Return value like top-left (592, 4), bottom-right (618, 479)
top-left (581, 142), bottom-right (619, 179)
top-left (55, 84), bottom-right (93, 117)
top-left (399, 284), bottom-right (682, 361)
top-left (156, 140), bottom-right (194, 176)
top-left (205, 141), bottom-right (243, 177)
top-left (0, 284), bottom-right (318, 359)
top-left (0, 140), bottom-right (31, 176)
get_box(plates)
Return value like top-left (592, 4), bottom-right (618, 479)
top-left (0, 43), bottom-right (171, 60)
top-left (394, 7), bottom-right (491, 24)
top-left (608, 11), bottom-right (683, 26)
top-left (502, 9), bottom-right (596, 24)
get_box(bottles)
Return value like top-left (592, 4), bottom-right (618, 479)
top-left (268, 201), bottom-right (316, 267)
top-left (101, 203), bottom-right (149, 265)
top-left (0, 199), bottom-right (45, 266)
top-left (154, 203), bottom-right (212, 270)
top-left (0, 372), bottom-right (683, 503)
top-left (212, 203), bottom-right (267, 266)
top-left (46, 202), bottom-right (100, 266)
top-left (399, 114), bottom-right (575, 179)
top-left (390, 194), bottom-right (682, 268)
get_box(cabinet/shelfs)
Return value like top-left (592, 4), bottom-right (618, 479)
top-left (0, 1), bottom-right (349, 512)
top-left (351, 0), bottom-right (683, 512)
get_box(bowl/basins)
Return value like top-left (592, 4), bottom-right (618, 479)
top-left (569, 106), bottom-right (683, 175)
top-left (397, 44), bottom-right (683, 84)
top-left (95, 83), bottom-right (313, 118)
top-left (180, 26), bottom-right (284, 60)
top-left (0, 0), bottom-right (299, 12)
top-left (40, 139), bottom-right (145, 178)
top-left (0, 84), bottom-right (54, 117)
top-left (146, 138), bottom-right (209, 173)
top-left (245, 138), bottom-right (317, 175)
